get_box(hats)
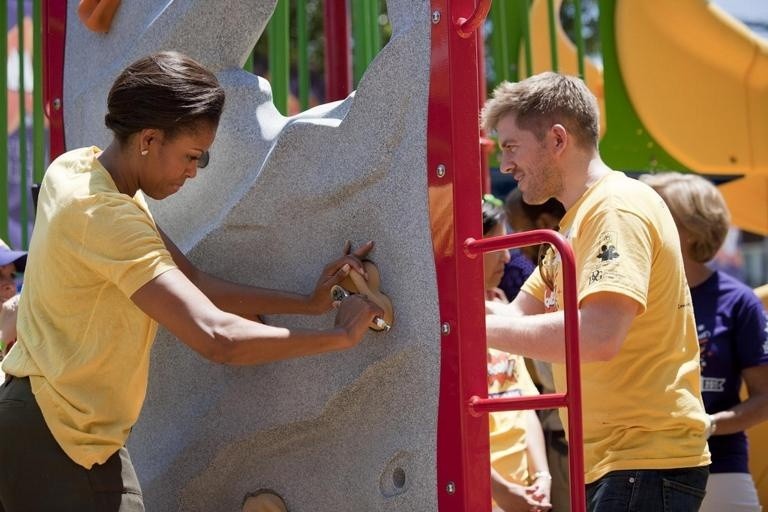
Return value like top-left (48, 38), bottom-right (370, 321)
top-left (0, 238), bottom-right (28, 273)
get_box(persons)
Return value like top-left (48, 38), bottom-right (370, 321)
top-left (639, 170), bottom-right (768, 512)
top-left (0, 47), bottom-right (384, 511)
top-left (480, 199), bottom-right (553, 512)
top-left (497, 185), bottom-right (570, 512)
top-left (1, 236), bottom-right (27, 365)
top-left (511, 230), bottom-right (574, 451)
top-left (477, 70), bottom-right (712, 512)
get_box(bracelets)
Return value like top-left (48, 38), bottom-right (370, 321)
top-left (529, 470), bottom-right (553, 484)
